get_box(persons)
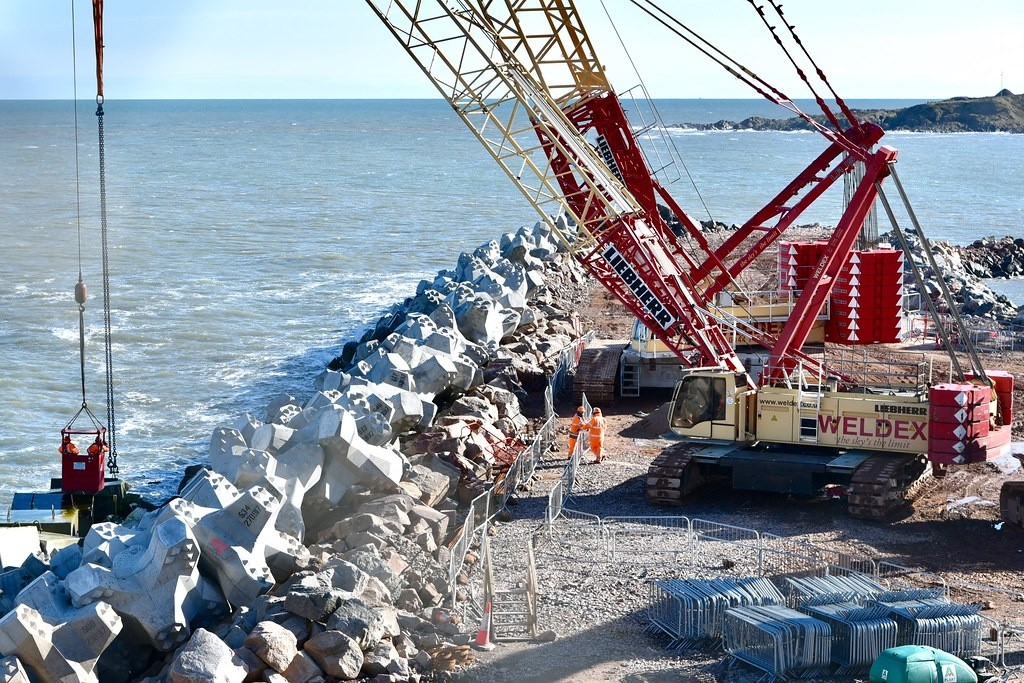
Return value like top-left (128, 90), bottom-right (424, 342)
top-left (568, 406), bottom-right (607, 464)
top-left (87, 436), bottom-right (110, 456)
top-left (58, 436), bottom-right (79, 455)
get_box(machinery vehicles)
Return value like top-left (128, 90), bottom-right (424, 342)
top-left (46, 0), bottom-right (1019, 533)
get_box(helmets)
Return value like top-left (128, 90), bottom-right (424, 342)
top-left (95, 436), bottom-right (101, 441)
top-left (65, 436), bottom-right (71, 442)
top-left (577, 406), bottom-right (586, 413)
top-left (594, 407), bottom-right (601, 413)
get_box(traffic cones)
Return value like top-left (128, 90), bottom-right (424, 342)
top-left (468, 601), bottom-right (495, 651)
